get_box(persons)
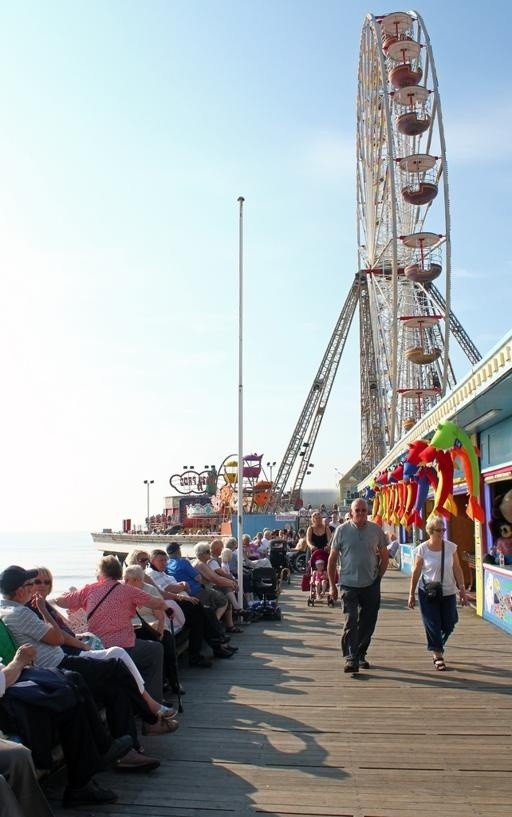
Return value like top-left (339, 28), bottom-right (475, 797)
top-left (386, 534), bottom-right (399, 559)
top-left (0, 556), bottom-right (180, 816)
top-left (125, 511), bottom-right (352, 696)
top-left (327, 498), bottom-right (389, 673)
top-left (408, 517), bottom-right (469, 670)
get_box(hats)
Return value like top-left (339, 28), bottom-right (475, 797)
top-left (0, 566), bottom-right (39, 594)
top-left (167, 542), bottom-right (182, 554)
top-left (315, 560), bottom-right (325, 564)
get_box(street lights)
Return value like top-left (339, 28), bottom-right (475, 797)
top-left (183, 466), bottom-right (194, 497)
top-left (144, 480), bottom-right (154, 525)
top-left (266, 462), bottom-right (276, 482)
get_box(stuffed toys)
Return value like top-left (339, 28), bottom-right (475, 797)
top-left (363, 439), bottom-right (454, 531)
top-left (429, 420), bottom-right (485, 524)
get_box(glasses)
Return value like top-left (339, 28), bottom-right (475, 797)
top-left (140, 557), bottom-right (149, 563)
top-left (34, 578), bottom-right (51, 585)
top-left (433, 528), bottom-right (446, 532)
top-left (354, 509), bottom-right (366, 513)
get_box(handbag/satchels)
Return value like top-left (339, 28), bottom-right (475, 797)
top-left (424, 582), bottom-right (442, 601)
top-left (6, 665), bottom-right (78, 710)
top-left (76, 632), bottom-right (104, 650)
top-left (134, 619), bottom-right (161, 642)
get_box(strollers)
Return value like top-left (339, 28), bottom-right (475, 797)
top-left (307, 547), bottom-right (336, 608)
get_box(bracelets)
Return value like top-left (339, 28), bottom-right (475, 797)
top-left (459, 584), bottom-right (465, 591)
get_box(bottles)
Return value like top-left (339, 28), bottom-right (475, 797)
top-left (500, 551), bottom-right (504, 568)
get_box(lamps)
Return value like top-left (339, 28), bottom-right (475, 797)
top-left (462, 408), bottom-right (502, 432)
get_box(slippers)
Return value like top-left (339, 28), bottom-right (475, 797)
top-left (154, 704), bottom-right (178, 719)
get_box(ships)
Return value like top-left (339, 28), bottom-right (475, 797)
top-left (89, 453), bottom-right (315, 544)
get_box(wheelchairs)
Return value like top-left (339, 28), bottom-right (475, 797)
top-left (289, 549), bottom-right (311, 573)
top-left (247, 567), bottom-right (282, 620)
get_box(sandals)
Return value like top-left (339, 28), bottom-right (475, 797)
top-left (433, 657), bottom-right (446, 671)
top-left (226, 626), bottom-right (243, 633)
top-left (233, 609), bottom-right (245, 616)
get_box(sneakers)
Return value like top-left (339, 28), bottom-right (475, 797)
top-left (189, 634), bottom-right (238, 668)
top-left (344, 653), bottom-right (369, 673)
top-left (163, 702), bottom-right (173, 707)
top-left (171, 682), bottom-right (185, 694)
top-left (62, 779), bottom-right (117, 806)
top-left (115, 747), bottom-right (160, 774)
